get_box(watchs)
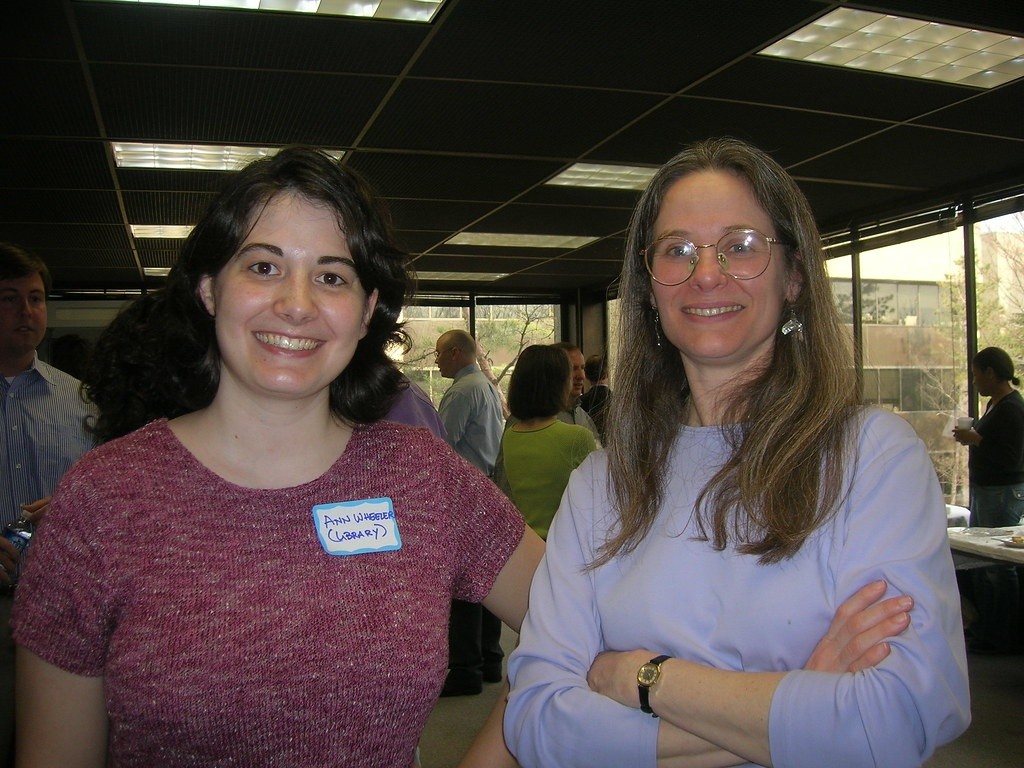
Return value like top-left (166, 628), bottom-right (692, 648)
top-left (637, 655), bottom-right (671, 719)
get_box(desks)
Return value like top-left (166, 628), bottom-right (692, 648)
top-left (946, 526), bottom-right (1024, 654)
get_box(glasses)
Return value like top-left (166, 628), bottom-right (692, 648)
top-left (434, 348), bottom-right (462, 359)
top-left (638, 228), bottom-right (794, 286)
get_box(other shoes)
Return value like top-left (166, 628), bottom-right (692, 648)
top-left (440, 669), bottom-right (483, 697)
top-left (964, 625), bottom-right (1018, 654)
top-left (480, 660), bottom-right (503, 683)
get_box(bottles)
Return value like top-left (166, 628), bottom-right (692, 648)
top-left (0, 509), bottom-right (34, 572)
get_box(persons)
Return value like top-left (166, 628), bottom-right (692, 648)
top-left (0, 242), bottom-right (101, 768)
top-left (384, 380), bottom-right (448, 439)
top-left (433, 329), bottom-right (506, 698)
top-left (493, 342), bottom-right (612, 543)
top-left (500, 135), bottom-right (972, 768)
top-left (952, 345), bottom-right (1024, 654)
top-left (13, 144), bottom-right (545, 768)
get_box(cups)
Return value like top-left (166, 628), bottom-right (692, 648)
top-left (957, 417), bottom-right (974, 430)
